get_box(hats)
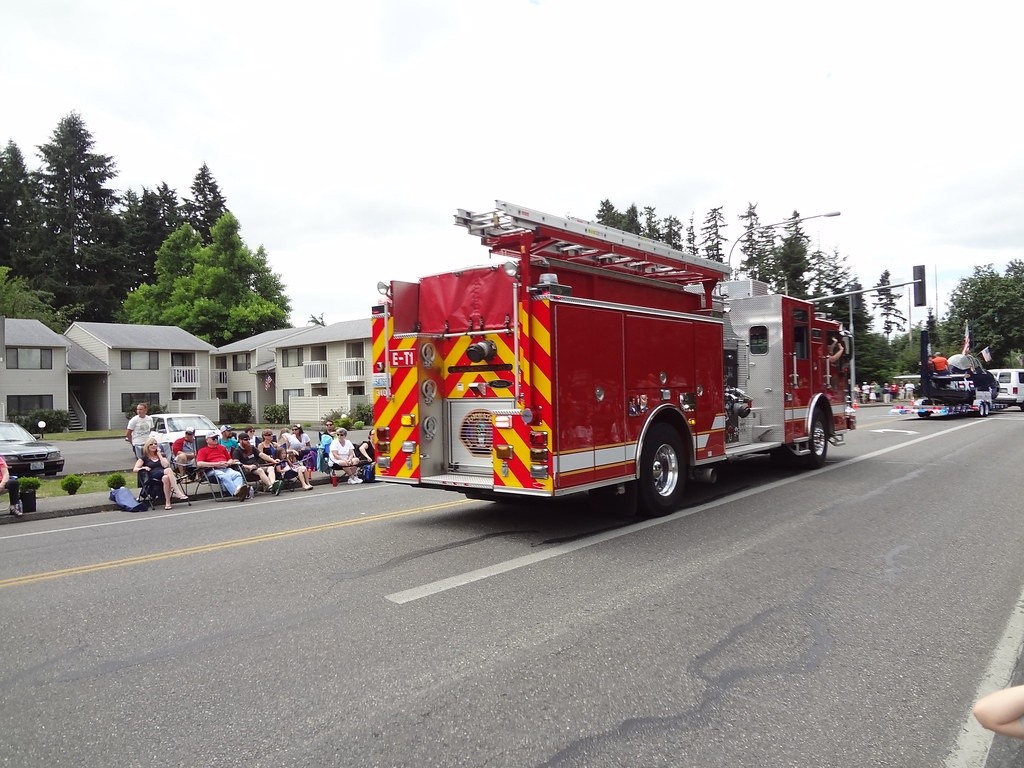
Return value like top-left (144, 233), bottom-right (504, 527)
top-left (863, 382), bottom-right (867, 385)
top-left (220, 425), bottom-right (235, 433)
top-left (186, 427), bottom-right (195, 434)
top-left (206, 430), bottom-right (219, 440)
top-left (933, 352), bottom-right (941, 356)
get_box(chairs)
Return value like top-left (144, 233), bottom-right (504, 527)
top-left (135, 432), bottom-right (375, 511)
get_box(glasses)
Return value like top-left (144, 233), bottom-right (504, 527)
top-left (292, 428), bottom-right (301, 431)
top-left (186, 432), bottom-right (193, 436)
top-left (241, 438), bottom-right (250, 441)
top-left (208, 436), bottom-right (218, 440)
top-left (326, 424), bottom-right (333, 427)
top-left (149, 443), bottom-right (157, 446)
top-left (337, 433), bottom-right (345, 436)
top-left (264, 434), bottom-right (273, 437)
top-left (247, 430), bottom-right (255, 433)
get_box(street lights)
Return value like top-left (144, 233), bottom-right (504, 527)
top-left (728, 208), bottom-right (842, 272)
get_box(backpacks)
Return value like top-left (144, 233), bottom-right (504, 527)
top-left (358, 463), bottom-right (375, 483)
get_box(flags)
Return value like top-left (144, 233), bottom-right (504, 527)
top-left (264, 372), bottom-right (273, 391)
top-left (981, 349), bottom-right (992, 363)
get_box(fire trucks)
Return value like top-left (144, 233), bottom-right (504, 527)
top-left (370, 198), bottom-right (857, 522)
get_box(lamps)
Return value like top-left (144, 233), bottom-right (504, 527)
top-left (38, 421), bottom-right (46, 428)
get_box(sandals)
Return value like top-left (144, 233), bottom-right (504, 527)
top-left (9, 508), bottom-right (24, 517)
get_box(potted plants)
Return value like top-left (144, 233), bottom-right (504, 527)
top-left (18, 476), bottom-right (43, 513)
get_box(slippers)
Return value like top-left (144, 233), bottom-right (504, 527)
top-left (176, 495), bottom-right (189, 501)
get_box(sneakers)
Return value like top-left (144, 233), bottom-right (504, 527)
top-left (347, 478), bottom-right (356, 485)
top-left (354, 477), bottom-right (363, 483)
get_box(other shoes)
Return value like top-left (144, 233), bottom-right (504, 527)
top-left (276, 480), bottom-right (283, 496)
top-left (269, 479), bottom-right (279, 494)
top-left (181, 478), bottom-right (186, 484)
top-left (165, 505), bottom-right (172, 510)
top-left (236, 485), bottom-right (250, 502)
top-left (302, 484), bottom-right (314, 491)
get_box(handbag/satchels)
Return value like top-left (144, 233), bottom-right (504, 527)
top-left (109, 487), bottom-right (149, 512)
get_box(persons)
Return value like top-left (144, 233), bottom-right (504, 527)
top-left (827, 336), bottom-right (844, 362)
top-left (132, 438), bottom-right (188, 509)
top-left (359, 428), bottom-right (375, 462)
top-left (972, 683), bottom-right (1024, 740)
top-left (855, 381), bottom-right (877, 405)
top-left (233, 433), bottom-right (284, 494)
top-left (905, 380), bottom-right (916, 400)
top-left (883, 380), bottom-right (906, 404)
top-left (932, 352), bottom-right (951, 375)
top-left (195, 430), bottom-right (251, 502)
top-left (0, 456), bottom-right (23, 516)
top-left (126, 404), bottom-right (154, 459)
top-left (259, 422), bottom-right (313, 490)
top-left (327, 428), bottom-right (364, 485)
top-left (324, 420), bottom-right (338, 437)
top-left (172, 427), bottom-right (203, 484)
top-left (217, 425), bottom-right (239, 453)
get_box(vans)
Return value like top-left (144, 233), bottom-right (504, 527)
top-left (986, 369), bottom-right (1024, 411)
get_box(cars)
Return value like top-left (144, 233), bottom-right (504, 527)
top-left (125, 413), bottom-right (224, 458)
top-left (0, 422), bottom-right (65, 477)
